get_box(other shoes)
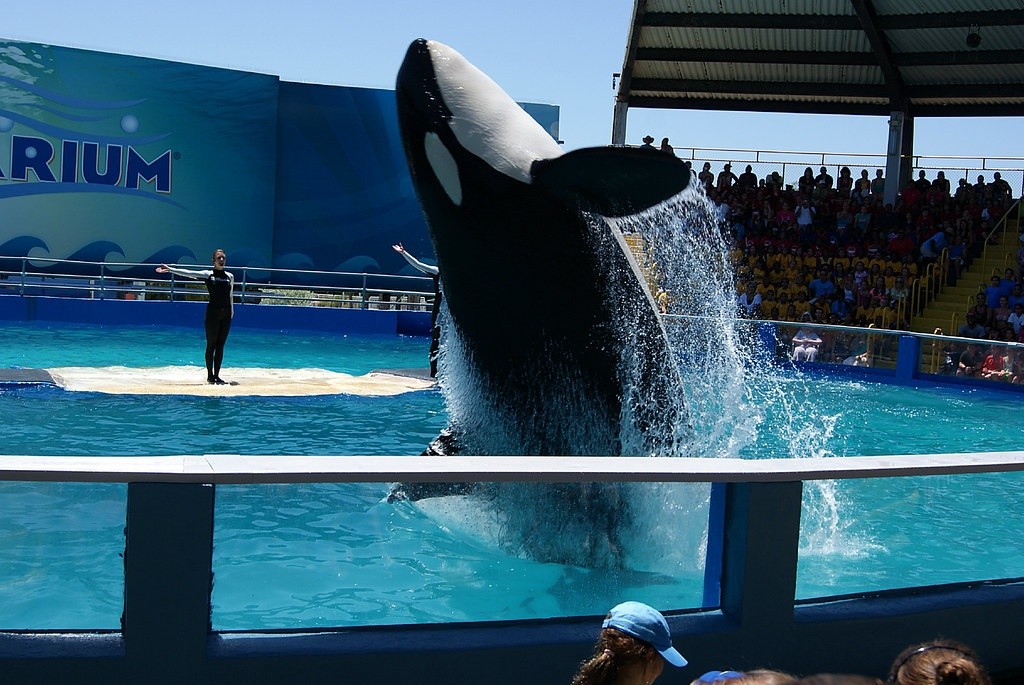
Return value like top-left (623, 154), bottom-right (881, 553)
top-left (214, 375), bottom-right (224, 382)
top-left (207, 376), bottom-right (215, 384)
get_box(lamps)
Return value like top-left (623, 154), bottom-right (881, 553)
top-left (967, 24), bottom-right (982, 47)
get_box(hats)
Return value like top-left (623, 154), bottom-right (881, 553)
top-left (601, 600), bottom-right (689, 668)
top-left (945, 227), bottom-right (954, 236)
top-left (699, 670), bottom-right (743, 685)
top-left (819, 180), bottom-right (825, 184)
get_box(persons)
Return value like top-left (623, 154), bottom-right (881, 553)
top-left (685, 160), bottom-right (1024, 384)
top-left (155, 248), bottom-right (235, 384)
top-left (392, 242), bottom-right (444, 378)
top-left (660, 137), bottom-right (674, 155)
top-left (887, 638), bottom-right (990, 685)
top-left (568, 601), bottom-right (673, 685)
top-left (690, 669), bottom-right (884, 685)
top-left (640, 135), bottom-right (656, 148)
